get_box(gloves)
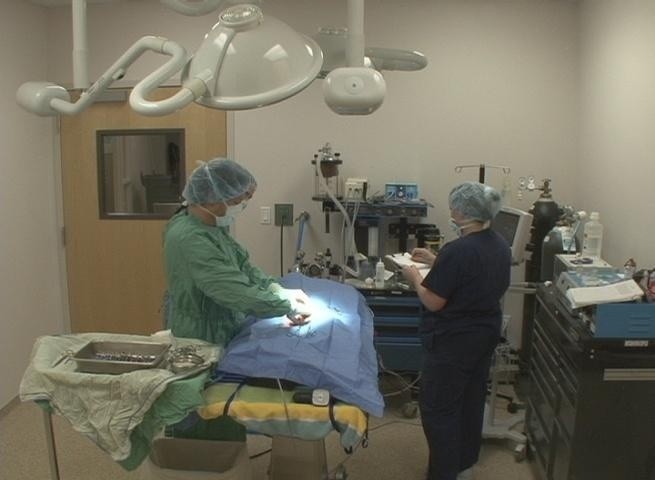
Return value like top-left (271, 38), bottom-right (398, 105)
top-left (267, 282), bottom-right (311, 306)
top-left (286, 306), bottom-right (313, 325)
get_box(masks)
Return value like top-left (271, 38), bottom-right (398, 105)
top-left (194, 160), bottom-right (248, 228)
top-left (448, 217), bottom-right (487, 238)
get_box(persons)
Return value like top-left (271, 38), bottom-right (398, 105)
top-left (399, 182), bottom-right (511, 479)
top-left (160, 157), bottom-right (309, 439)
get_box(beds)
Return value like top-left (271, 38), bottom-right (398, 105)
top-left (194, 273), bottom-right (369, 480)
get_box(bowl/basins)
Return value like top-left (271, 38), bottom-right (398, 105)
top-left (168, 353), bottom-right (205, 374)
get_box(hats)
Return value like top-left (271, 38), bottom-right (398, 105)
top-left (181, 158), bottom-right (257, 206)
top-left (447, 181), bottom-right (501, 221)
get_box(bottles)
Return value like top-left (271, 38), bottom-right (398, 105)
top-left (406, 234), bottom-right (418, 254)
top-left (582, 212), bottom-right (603, 261)
top-left (375, 256), bottom-right (385, 289)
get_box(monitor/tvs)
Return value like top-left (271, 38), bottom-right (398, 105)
top-left (490, 206), bottom-right (534, 265)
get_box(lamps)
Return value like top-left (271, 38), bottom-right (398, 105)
top-left (180, 2), bottom-right (324, 111)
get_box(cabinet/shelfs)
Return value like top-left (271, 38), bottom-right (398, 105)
top-left (523, 282), bottom-right (655, 480)
top-left (336, 286), bottom-right (424, 376)
